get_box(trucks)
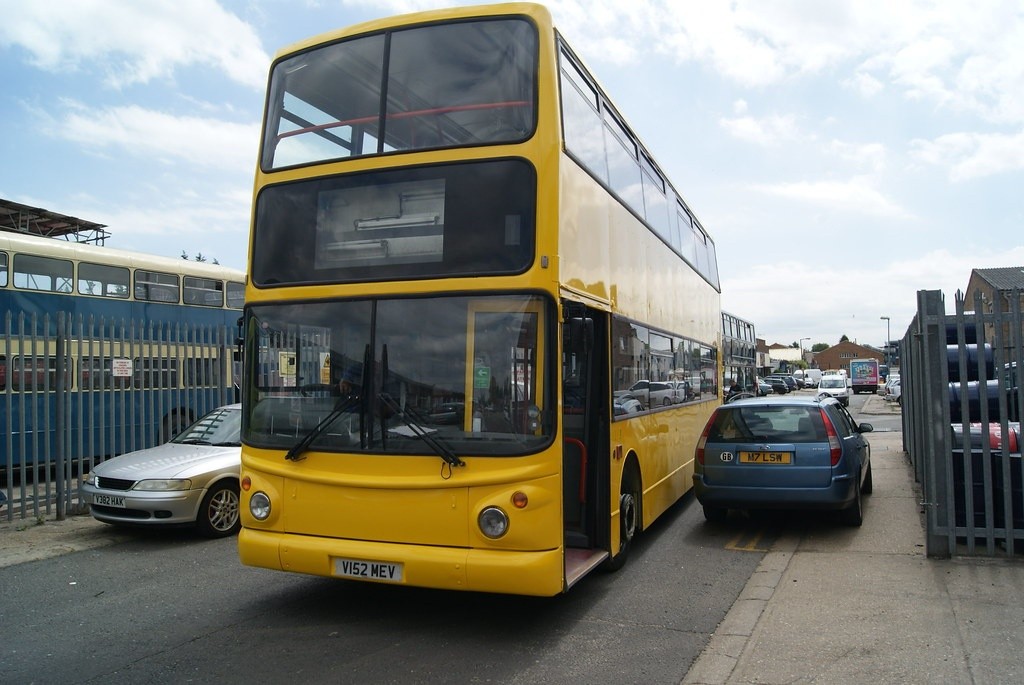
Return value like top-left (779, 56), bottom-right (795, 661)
top-left (850, 358), bottom-right (879, 394)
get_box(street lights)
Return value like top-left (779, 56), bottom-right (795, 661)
top-left (881, 317), bottom-right (890, 379)
top-left (800, 338), bottom-right (811, 379)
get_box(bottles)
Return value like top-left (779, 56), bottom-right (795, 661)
top-left (473, 410), bottom-right (484, 432)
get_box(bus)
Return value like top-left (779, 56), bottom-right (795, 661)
top-left (234, 0), bottom-right (724, 601)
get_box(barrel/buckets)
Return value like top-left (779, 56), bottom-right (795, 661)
top-left (951, 427), bottom-right (1021, 453)
top-left (951, 422), bottom-right (1020, 427)
top-left (945, 311), bottom-right (985, 344)
top-left (952, 453), bottom-right (997, 546)
top-left (995, 453), bottom-right (1024, 554)
top-left (946, 343), bottom-right (994, 381)
top-left (949, 379), bottom-right (1000, 422)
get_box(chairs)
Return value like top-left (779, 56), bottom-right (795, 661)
top-left (759, 418), bottom-right (773, 429)
top-left (798, 418), bottom-right (810, 432)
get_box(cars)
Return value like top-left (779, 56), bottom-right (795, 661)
top-left (78, 403), bottom-right (245, 538)
top-left (805, 378), bottom-right (814, 387)
top-left (796, 379), bottom-right (806, 390)
top-left (877, 378), bottom-right (901, 404)
top-left (617, 380), bottom-right (685, 407)
top-left (758, 383), bottom-right (774, 396)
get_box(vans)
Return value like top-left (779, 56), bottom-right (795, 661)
top-left (685, 377), bottom-right (700, 395)
top-left (793, 369), bottom-right (821, 389)
top-left (819, 376), bottom-right (851, 405)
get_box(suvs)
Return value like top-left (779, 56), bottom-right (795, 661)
top-left (613, 394), bottom-right (643, 417)
top-left (694, 392), bottom-right (876, 527)
top-left (767, 376), bottom-right (798, 393)
top-left (765, 380), bottom-right (789, 394)
